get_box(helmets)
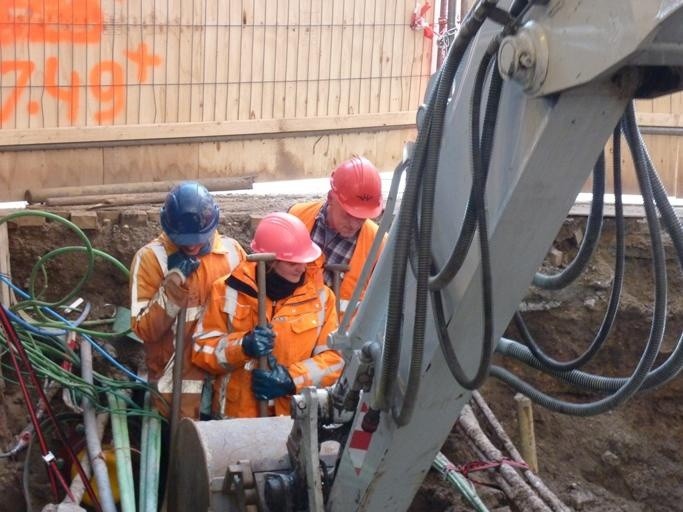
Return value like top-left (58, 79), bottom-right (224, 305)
top-left (159, 180), bottom-right (221, 248)
top-left (249, 210), bottom-right (325, 265)
top-left (329, 154), bottom-right (387, 222)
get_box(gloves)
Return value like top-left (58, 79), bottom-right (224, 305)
top-left (248, 355), bottom-right (296, 401)
top-left (240, 318), bottom-right (278, 360)
top-left (166, 252), bottom-right (202, 288)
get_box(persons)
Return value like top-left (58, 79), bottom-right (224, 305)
top-left (284, 154), bottom-right (390, 331)
top-left (127, 180), bottom-right (249, 422)
top-left (190, 210), bottom-right (343, 421)
